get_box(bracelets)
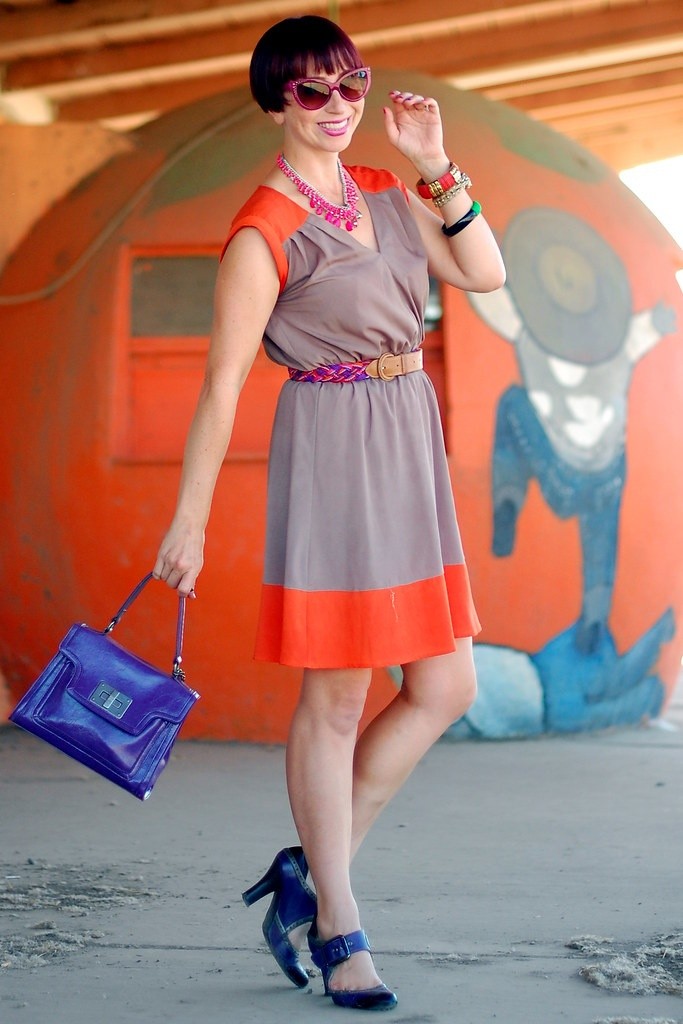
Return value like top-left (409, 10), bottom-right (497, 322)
top-left (433, 174), bottom-right (471, 208)
top-left (416, 162), bottom-right (462, 199)
top-left (442, 201), bottom-right (481, 237)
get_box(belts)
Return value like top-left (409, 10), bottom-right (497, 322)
top-left (288, 347), bottom-right (424, 383)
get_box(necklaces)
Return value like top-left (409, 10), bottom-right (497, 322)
top-left (277, 152), bottom-right (361, 230)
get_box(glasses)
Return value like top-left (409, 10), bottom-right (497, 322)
top-left (282, 67), bottom-right (372, 111)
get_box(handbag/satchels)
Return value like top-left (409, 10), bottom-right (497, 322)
top-left (8, 570), bottom-right (199, 800)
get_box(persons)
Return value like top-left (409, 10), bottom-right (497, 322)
top-left (154, 15), bottom-right (506, 1009)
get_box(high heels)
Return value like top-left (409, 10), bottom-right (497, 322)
top-left (242, 847), bottom-right (320, 989)
top-left (306, 915), bottom-right (398, 1012)
